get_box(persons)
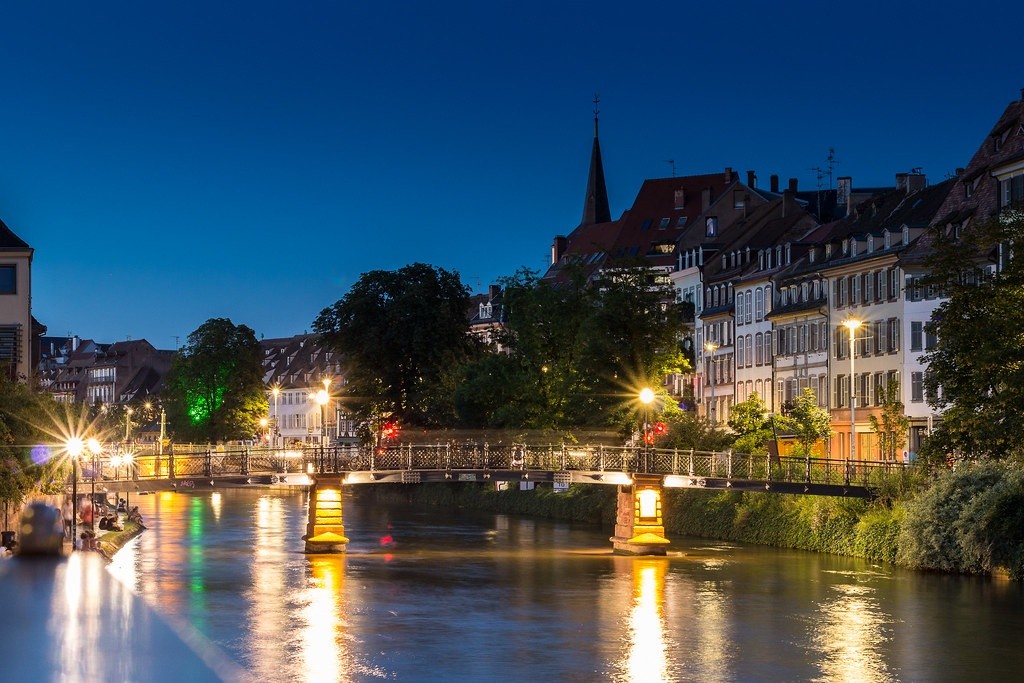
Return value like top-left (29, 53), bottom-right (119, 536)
top-left (55, 508), bottom-right (64, 555)
top-left (80, 529), bottom-right (114, 565)
top-left (82, 494), bottom-right (109, 531)
top-left (117, 499), bottom-right (142, 524)
top-left (60, 497), bottom-right (74, 540)
top-left (99, 514), bottom-right (123, 532)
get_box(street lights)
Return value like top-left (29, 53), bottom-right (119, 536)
top-left (272, 387), bottom-right (281, 446)
top-left (315, 389), bottom-right (330, 473)
top-left (69, 438), bottom-right (79, 550)
top-left (703, 340), bottom-right (720, 429)
top-left (124, 453), bottom-right (134, 520)
top-left (844, 316), bottom-right (861, 467)
top-left (638, 384), bottom-right (655, 471)
top-left (112, 457), bottom-right (121, 518)
top-left (88, 437), bottom-right (101, 531)
top-left (99, 402), bottom-right (153, 443)
